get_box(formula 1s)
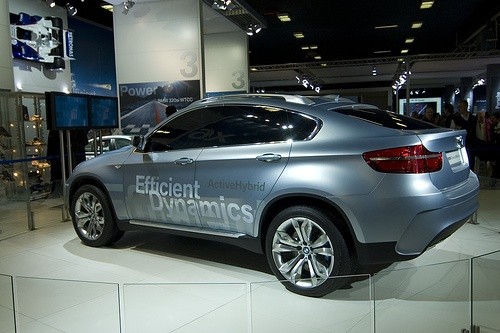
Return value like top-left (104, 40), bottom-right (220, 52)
top-left (10, 12), bottom-right (76, 72)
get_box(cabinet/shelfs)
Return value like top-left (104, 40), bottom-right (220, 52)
top-left (0, 92), bottom-right (54, 202)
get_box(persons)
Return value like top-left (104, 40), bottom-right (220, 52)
top-left (46, 127), bottom-right (90, 193)
top-left (405, 101), bottom-right (500, 181)
top-left (450, 100), bottom-right (477, 176)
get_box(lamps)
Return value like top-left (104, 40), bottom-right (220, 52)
top-left (212, 0), bottom-right (232, 11)
top-left (123, 0), bottom-right (135, 11)
top-left (246, 23), bottom-right (262, 36)
top-left (66, 2), bottom-right (77, 16)
top-left (41, 0), bottom-right (55, 8)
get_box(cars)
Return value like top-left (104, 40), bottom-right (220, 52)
top-left (66, 93), bottom-right (481, 298)
top-left (85, 130), bottom-right (170, 161)
